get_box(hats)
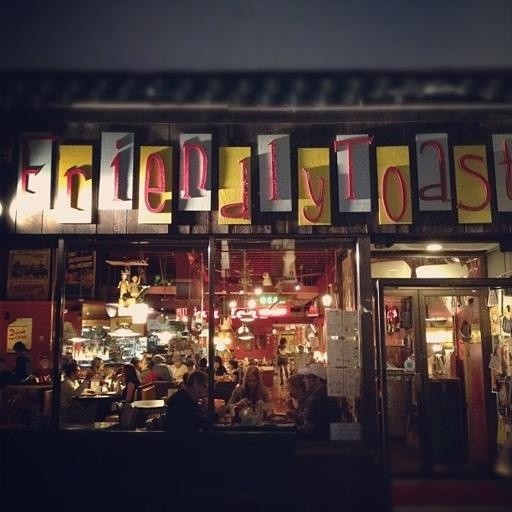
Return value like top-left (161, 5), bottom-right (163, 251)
top-left (297, 363), bottom-right (328, 380)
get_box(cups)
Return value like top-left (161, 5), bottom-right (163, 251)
top-left (224, 414), bottom-right (232, 426)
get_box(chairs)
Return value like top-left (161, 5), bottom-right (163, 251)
top-left (41, 388), bottom-right (54, 428)
top-left (316, 394), bottom-right (350, 431)
top-left (131, 380), bottom-right (179, 431)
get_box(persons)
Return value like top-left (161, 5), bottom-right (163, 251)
top-left (118, 273), bottom-right (131, 299)
top-left (128, 275), bottom-right (142, 299)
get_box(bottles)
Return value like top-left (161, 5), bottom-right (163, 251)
top-left (404, 356), bottom-right (414, 370)
top-left (234, 399), bottom-right (264, 427)
top-left (82, 377), bottom-right (121, 396)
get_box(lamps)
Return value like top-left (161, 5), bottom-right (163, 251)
top-left (84, 262), bottom-right (335, 353)
